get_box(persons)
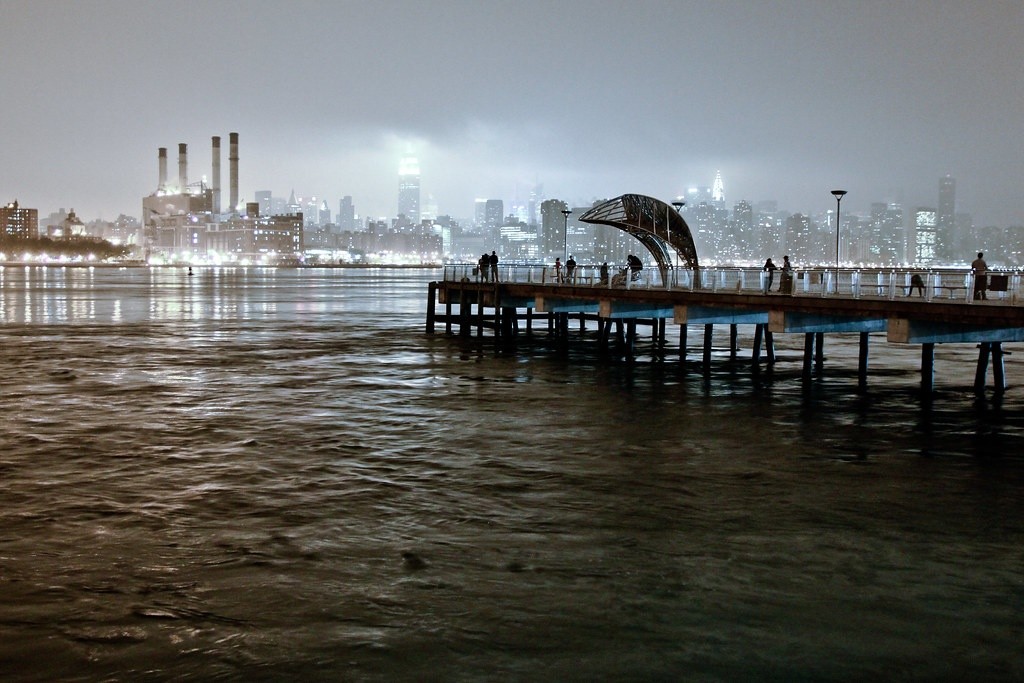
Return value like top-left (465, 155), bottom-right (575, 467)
top-left (627, 255), bottom-right (643, 272)
top-left (567, 256), bottom-right (576, 282)
top-left (909, 275), bottom-right (924, 296)
top-left (601, 262), bottom-right (609, 280)
top-left (777, 256), bottom-right (791, 292)
top-left (972, 252), bottom-right (989, 300)
top-left (764, 258), bottom-right (776, 291)
top-left (479, 251), bottom-right (499, 283)
top-left (555, 258), bottom-right (565, 283)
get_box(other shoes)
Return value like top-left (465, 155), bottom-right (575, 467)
top-left (981, 297), bottom-right (988, 300)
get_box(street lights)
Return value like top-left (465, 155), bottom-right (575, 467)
top-left (561, 210), bottom-right (572, 281)
top-left (672, 202), bottom-right (685, 288)
top-left (831, 190), bottom-right (848, 295)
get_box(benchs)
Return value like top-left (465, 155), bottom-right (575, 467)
top-left (860, 284), bottom-right (1015, 302)
top-left (550, 275), bottom-right (602, 285)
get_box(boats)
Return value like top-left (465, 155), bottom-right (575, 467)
top-left (188, 271), bottom-right (194, 275)
top-left (189, 267), bottom-right (192, 270)
